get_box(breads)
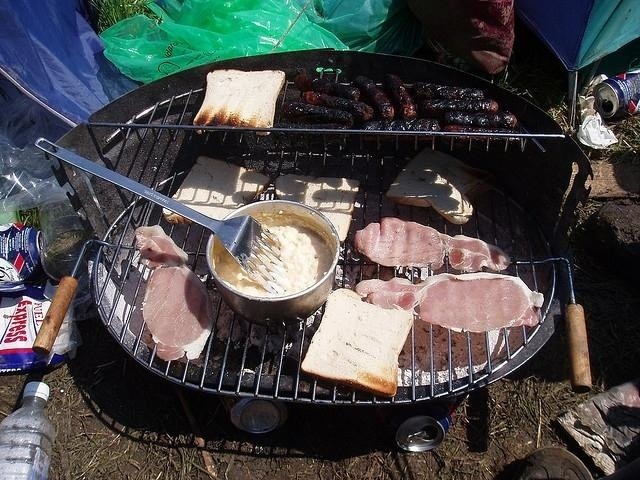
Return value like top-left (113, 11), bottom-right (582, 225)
top-left (299, 287), bottom-right (415, 397)
top-left (193, 69), bottom-right (287, 136)
top-left (273, 174), bottom-right (360, 247)
top-left (385, 148), bottom-right (499, 225)
top-left (161, 154), bottom-right (271, 228)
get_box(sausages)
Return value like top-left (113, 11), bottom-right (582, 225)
top-left (282, 70), bottom-right (522, 142)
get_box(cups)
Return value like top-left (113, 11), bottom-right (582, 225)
top-left (40, 193), bottom-right (92, 282)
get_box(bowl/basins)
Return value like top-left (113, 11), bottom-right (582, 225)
top-left (205, 200), bottom-right (340, 326)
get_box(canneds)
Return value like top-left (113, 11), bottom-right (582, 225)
top-left (0, 222), bottom-right (47, 293)
top-left (224, 397), bottom-right (293, 434)
top-left (392, 400), bottom-right (458, 453)
top-left (593, 68), bottom-right (640, 120)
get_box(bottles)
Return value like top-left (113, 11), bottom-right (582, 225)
top-left (1, 381), bottom-right (58, 480)
